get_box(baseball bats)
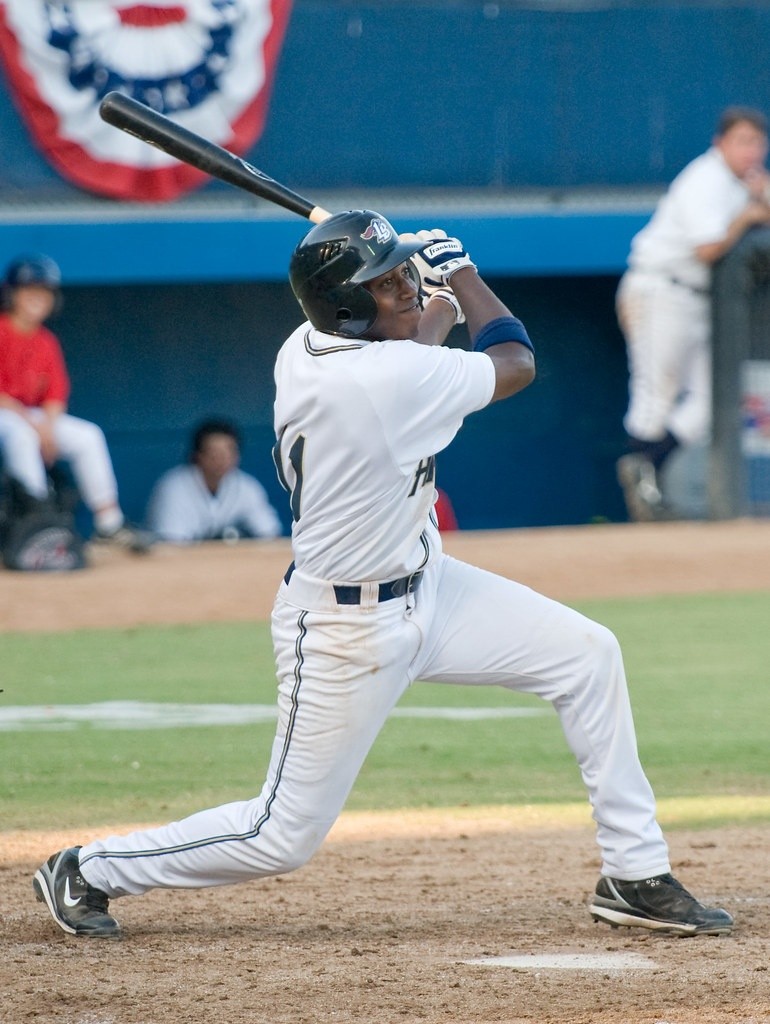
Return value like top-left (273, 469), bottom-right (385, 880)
top-left (98, 89), bottom-right (336, 227)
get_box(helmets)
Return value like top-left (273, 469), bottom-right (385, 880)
top-left (288, 209), bottom-right (434, 339)
top-left (4, 254), bottom-right (65, 316)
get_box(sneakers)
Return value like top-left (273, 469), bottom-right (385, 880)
top-left (588, 873), bottom-right (734, 935)
top-left (32, 844), bottom-right (121, 936)
top-left (92, 521), bottom-right (160, 554)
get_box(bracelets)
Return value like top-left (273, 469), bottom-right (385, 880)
top-left (471, 316), bottom-right (534, 357)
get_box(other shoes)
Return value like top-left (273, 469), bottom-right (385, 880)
top-left (617, 454), bottom-right (666, 522)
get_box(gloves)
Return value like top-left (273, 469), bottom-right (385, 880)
top-left (400, 228), bottom-right (477, 284)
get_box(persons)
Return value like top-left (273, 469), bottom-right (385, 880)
top-left (1, 256), bottom-right (143, 556)
top-left (614, 106), bottom-right (770, 526)
top-left (31, 210), bottom-right (737, 933)
top-left (144, 415), bottom-right (280, 541)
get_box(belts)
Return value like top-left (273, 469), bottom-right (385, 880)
top-left (284, 560), bottom-right (425, 605)
top-left (674, 278), bottom-right (711, 295)
